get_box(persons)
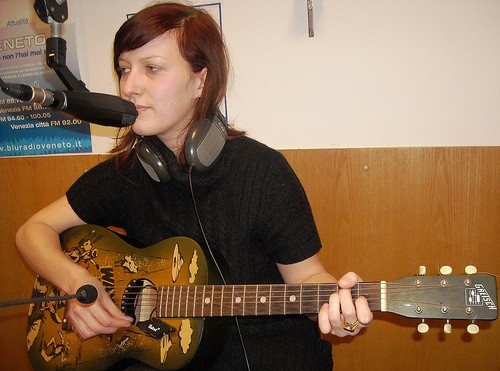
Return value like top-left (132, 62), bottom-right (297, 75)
top-left (15, 2), bottom-right (374, 371)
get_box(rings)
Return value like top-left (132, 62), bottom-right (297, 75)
top-left (342, 319), bottom-right (359, 332)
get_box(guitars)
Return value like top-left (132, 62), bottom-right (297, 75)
top-left (26, 224), bottom-right (498, 371)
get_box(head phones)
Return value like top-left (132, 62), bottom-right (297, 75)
top-left (135, 108), bottom-right (227, 184)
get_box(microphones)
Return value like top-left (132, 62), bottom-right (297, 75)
top-left (0, 285), bottom-right (98, 307)
top-left (6, 83), bottom-right (139, 128)
